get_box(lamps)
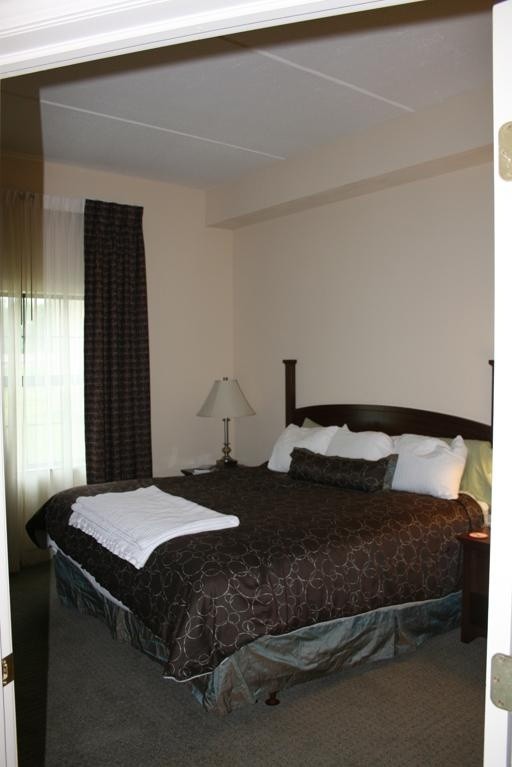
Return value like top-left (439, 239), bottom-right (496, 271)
top-left (195, 375), bottom-right (257, 470)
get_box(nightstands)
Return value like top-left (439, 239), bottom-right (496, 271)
top-left (454, 526), bottom-right (490, 644)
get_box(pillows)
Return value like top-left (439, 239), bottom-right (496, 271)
top-left (265, 421), bottom-right (470, 503)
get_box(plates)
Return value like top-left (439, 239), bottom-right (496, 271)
top-left (469, 532), bottom-right (488, 539)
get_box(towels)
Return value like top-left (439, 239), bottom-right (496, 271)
top-left (66, 484), bottom-right (241, 572)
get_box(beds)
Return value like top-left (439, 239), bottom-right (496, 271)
top-left (41, 356), bottom-right (492, 708)
